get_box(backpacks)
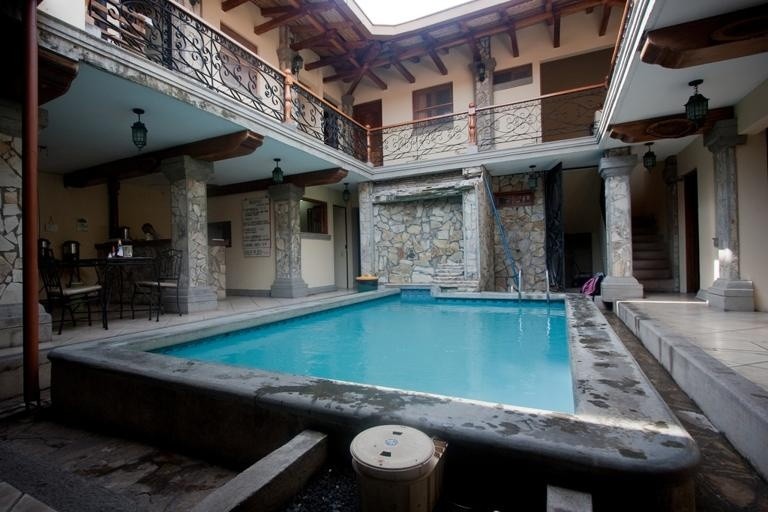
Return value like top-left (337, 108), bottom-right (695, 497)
top-left (582, 275), bottom-right (603, 294)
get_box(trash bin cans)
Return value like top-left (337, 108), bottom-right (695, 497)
top-left (356, 273), bottom-right (379, 293)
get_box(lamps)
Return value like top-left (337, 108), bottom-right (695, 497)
top-left (130, 109), bottom-right (148, 152)
top-left (683, 79), bottom-right (709, 129)
top-left (273, 159), bottom-right (283, 184)
top-left (292, 56), bottom-right (303, 74)
top-left (342, 183), bottom-right (351, 202)
top-left (643, 143), bottom-right (656, 173)
top-left (528, 165), bottom-right (537, 192)
top-left (478, 62), bottom-right (486, 81)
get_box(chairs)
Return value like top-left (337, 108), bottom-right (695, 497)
top-left (38, 248), bottom-right (183, 334)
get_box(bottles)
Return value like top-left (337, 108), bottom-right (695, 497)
top-left (116, 239), bottom-right (124, 259)
